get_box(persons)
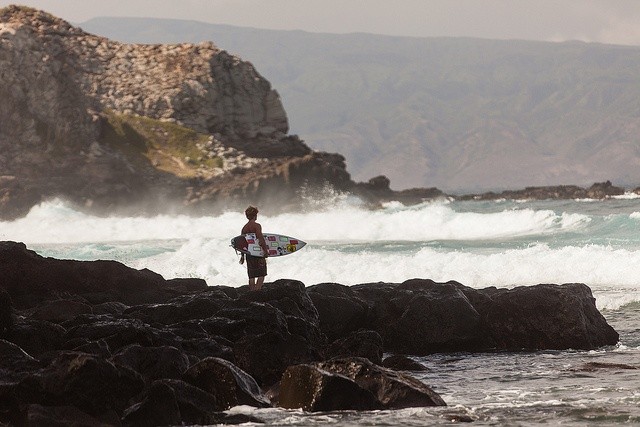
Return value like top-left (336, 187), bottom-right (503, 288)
top-left (239, 207), bottom-right (268, 291)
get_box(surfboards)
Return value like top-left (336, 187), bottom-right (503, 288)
top-left (231, 233), bottom-right (307, 257)
